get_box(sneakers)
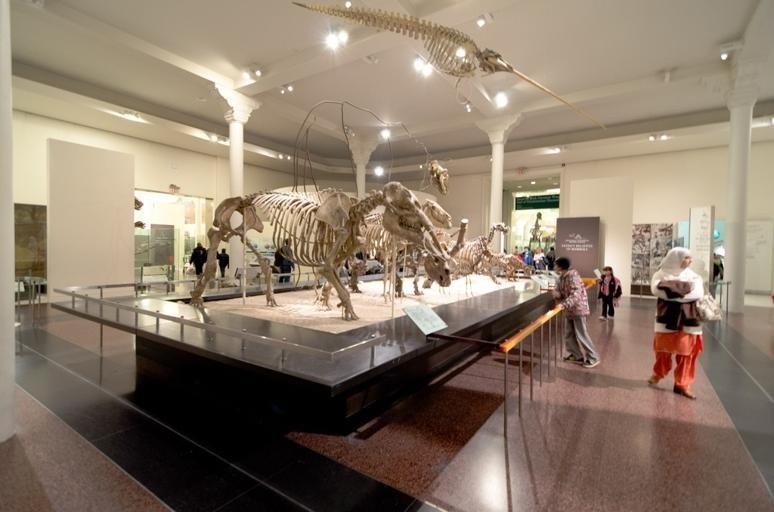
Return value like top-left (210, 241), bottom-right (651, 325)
top-left (649, 371), bottom-right (664, 388)
top-left (562, 353), bottom-right (586, 365)
top-left (673, 384), bottom-right (697, 402)
top-left (583, 357), bottom-right (601, 368)
top-left (599, 315), bottom-right (614, 321)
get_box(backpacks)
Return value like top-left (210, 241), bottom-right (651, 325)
top-left (614, 277), bottom-right (622, 298)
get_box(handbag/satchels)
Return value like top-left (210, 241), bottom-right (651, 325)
top-left (694, 293), bottom-right (722, 323)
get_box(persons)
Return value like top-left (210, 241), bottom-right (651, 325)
top-left (600, 266), bottom-right (622, 321)
top-left (646, 246), bottom-right (708, 397)
top-left (218, 248), bottom-right (230, 278)
top-left (190, 243), bottom-right (208, 277)
top-left (515, 248), bottom-right (555, 271)
top-left (274, 239), bottom-right (297, 283)
top-left (554, 256), bottom-right (599, 368)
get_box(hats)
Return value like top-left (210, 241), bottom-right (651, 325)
top-left (555, 257), bottom-right (571, 269)
